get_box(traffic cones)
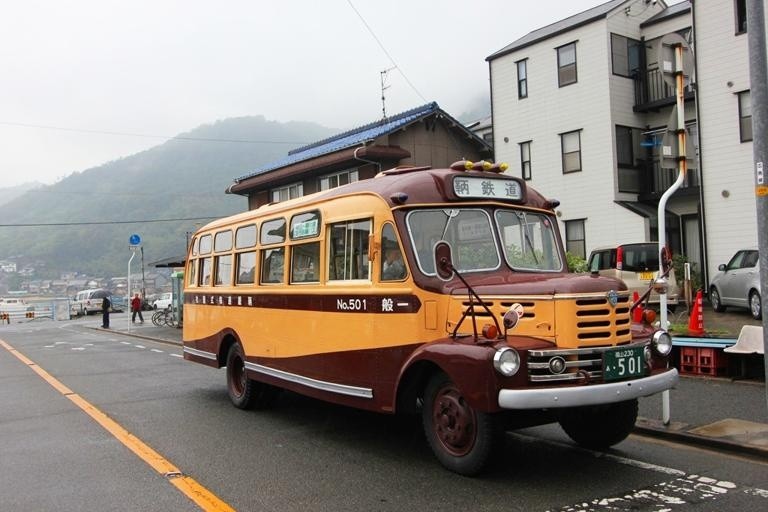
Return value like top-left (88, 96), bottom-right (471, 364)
top-left (686, 290), bottom-right (704, 333)
top-left (631, 291), bottom-right (643, 324)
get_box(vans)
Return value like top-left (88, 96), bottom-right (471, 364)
top-left (587, 242), bottom-right (679, 314)
top-left (71, 289), bottom-right (113, 315)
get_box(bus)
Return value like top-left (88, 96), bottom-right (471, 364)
top-left (180, 161), bottom-right (679, 479)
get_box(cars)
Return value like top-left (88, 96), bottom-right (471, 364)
top-left (152, 292), bottom-right (177, 310)
top-left (709, 250), bottom-right (762, 320)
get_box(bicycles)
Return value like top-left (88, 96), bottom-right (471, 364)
top-left (151, 305), bottom-right (174, 328)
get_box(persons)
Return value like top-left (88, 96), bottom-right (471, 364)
top-left (101, 296), bottom-right (111, 329)
top-left (131, 293), bottom-right (144, 323)
top-left (381, 247), bottom-right (405, 280)
